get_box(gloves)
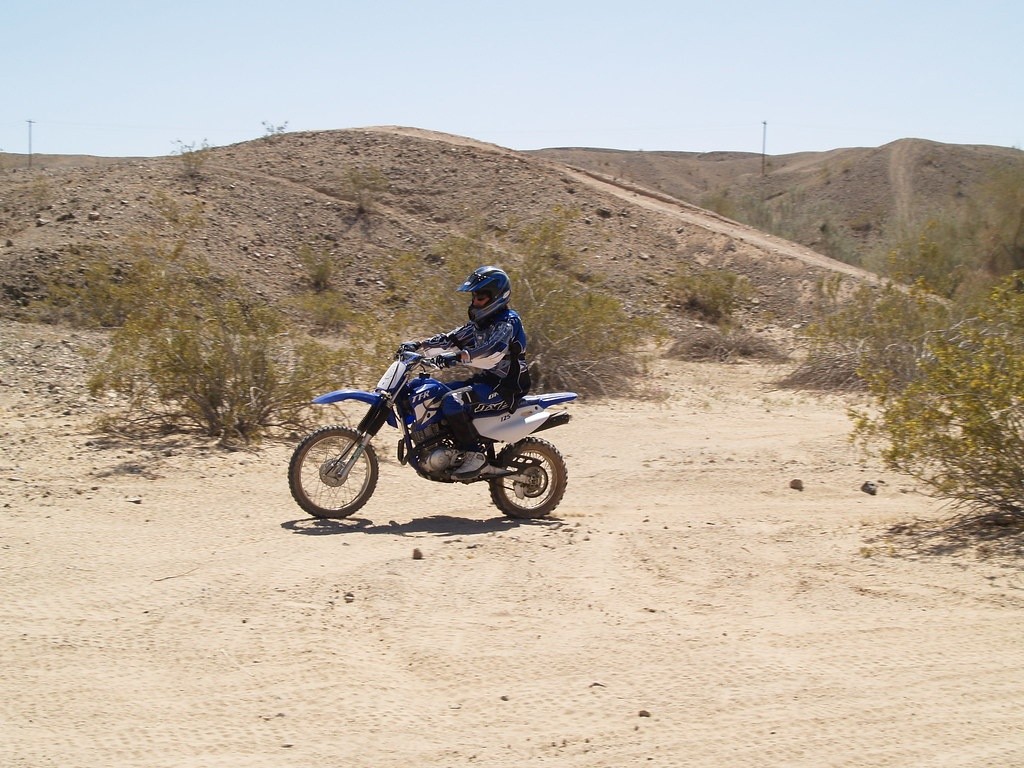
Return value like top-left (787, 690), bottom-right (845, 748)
top-left (394, 340), bottom-right (419, 359)
top-left (434, 351), bottom-right (460, 371)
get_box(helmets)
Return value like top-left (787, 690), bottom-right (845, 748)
top-left (455, 265), bottom-right (512, 322)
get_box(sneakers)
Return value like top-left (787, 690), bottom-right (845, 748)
top-left (451, 451), bottom-right (489, 479)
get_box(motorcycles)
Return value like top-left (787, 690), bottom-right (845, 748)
top-left (286, 342), bottom-right (580, 520)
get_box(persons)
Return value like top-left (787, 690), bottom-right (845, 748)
top-left (400, 265), bottom-right (530, 480)
